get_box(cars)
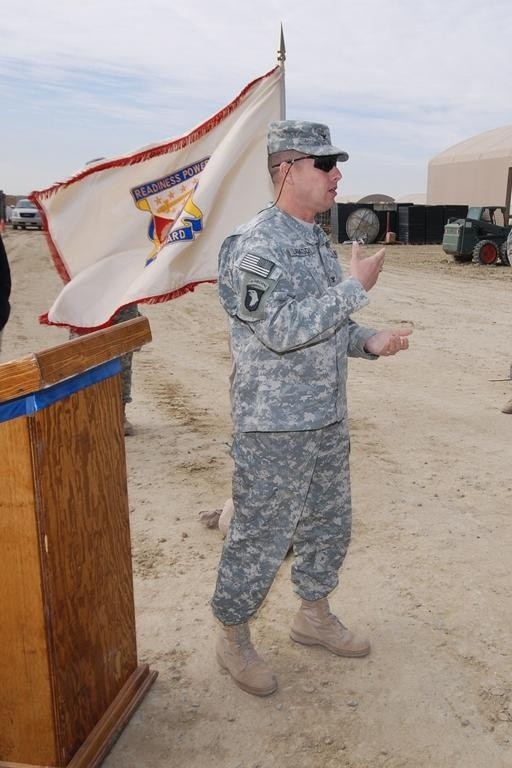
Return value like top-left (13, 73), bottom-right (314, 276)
top-left (11, 198), bottom-right (43, 230)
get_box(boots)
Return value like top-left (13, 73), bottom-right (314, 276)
top-left (214, 618), bottom-right (279, 697)
top-left (289, 597), bottom-right (371, 658)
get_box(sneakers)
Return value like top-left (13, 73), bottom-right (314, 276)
top-left (501, 399), bottom-right (512, 414)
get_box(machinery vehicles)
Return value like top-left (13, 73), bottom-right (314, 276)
top-left (443, 206), bottom-right (512, 266)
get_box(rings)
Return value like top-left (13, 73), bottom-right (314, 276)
top-left (387, 351), bottom-right (392, 354)
top-left (379, 267), bottom-right (383, 272)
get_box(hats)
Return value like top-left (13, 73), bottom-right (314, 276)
top-left (266, 118), bottom-right (349, 163)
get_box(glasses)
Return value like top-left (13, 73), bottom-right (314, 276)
top-left (271, 154), bottom-right (337, 173)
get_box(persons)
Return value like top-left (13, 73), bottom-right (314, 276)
top-left (68, 302), bottom-right (141, 438)
top-left (205, 119), bottom-right (414, 699)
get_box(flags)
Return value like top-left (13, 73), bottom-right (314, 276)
top-left (21, 60), bottom-right (282, 336)
